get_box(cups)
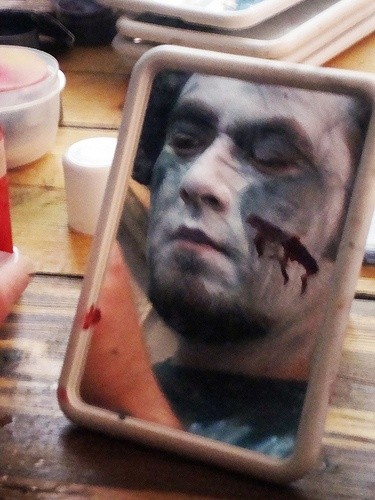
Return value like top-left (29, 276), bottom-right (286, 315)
top-left (62, 137), bottom-right (117, 235)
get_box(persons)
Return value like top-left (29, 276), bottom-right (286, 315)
top-left (78, 67), bottom-right (372, 464)
top-left (0, 252), bottom-right (34, 334)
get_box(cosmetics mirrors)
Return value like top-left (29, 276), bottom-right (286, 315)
top-left (94, 0), bottom-right (374, 67)
top-left (58, 44), bottom-right (375, 488)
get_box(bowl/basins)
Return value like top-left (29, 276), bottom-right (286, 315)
top-left (0, 44), bottom-right (66, 170)
